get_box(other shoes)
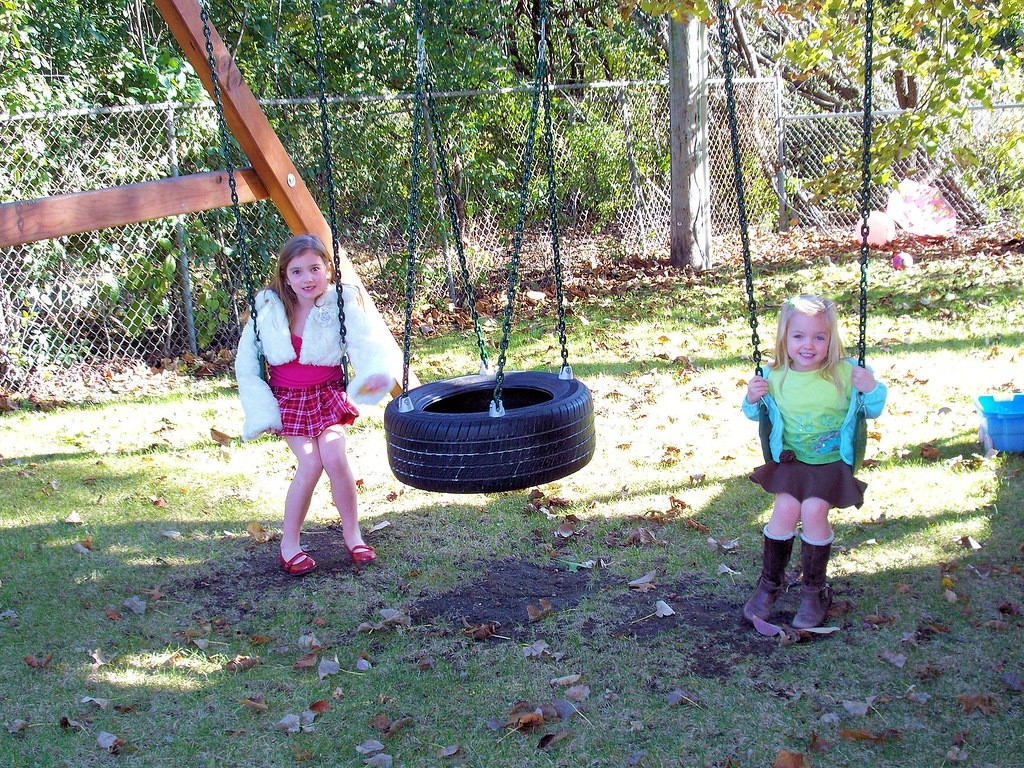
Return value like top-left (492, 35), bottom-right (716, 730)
top-left (280, 552), bottom-right (317, 575)
top-left (344, 543), bottom-right (376, 563)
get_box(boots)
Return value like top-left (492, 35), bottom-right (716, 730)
top-left (792, 530), bottom-right (834, 629)
top-left (744, 524), bottom-right (797, 623)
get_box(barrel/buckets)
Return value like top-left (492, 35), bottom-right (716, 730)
top-left (975, 393), bottom-right (1024, 454)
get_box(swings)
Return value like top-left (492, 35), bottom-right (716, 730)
top-left (198, 0), bottom-right (349, 393)
top-left (714, 2), bottom-right (875, 473)
top-left (381, 0), bottom-right (597, 493)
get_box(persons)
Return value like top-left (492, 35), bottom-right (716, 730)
top-left (741, 294), bottom-right (889, 631)
top-left (235, 235), bottom-right (395, 575)
